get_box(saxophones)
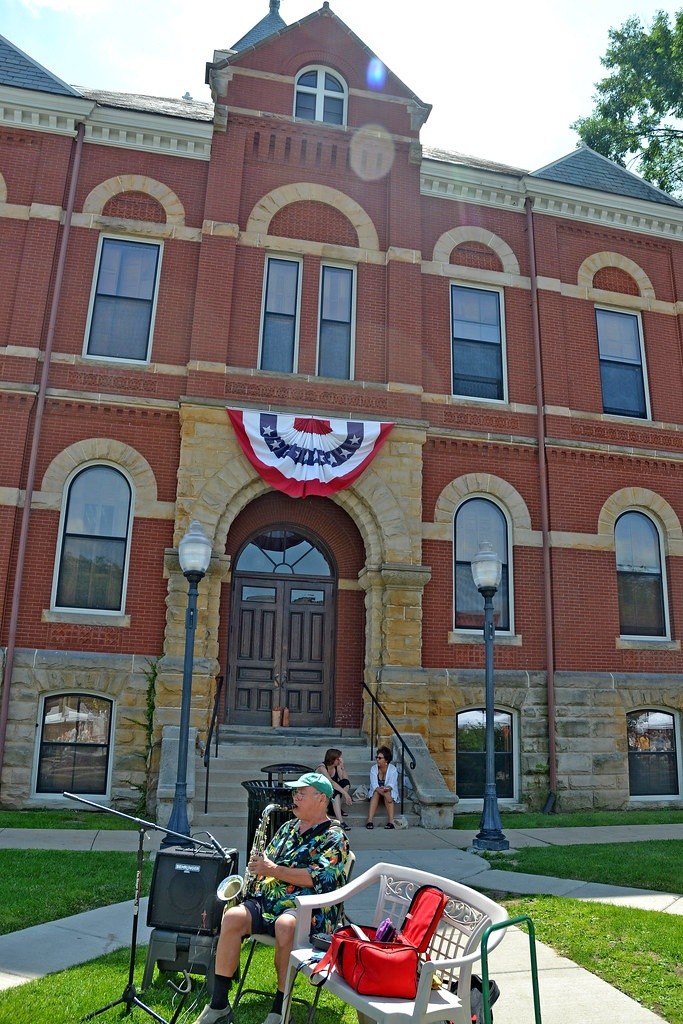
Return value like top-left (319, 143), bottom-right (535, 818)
top-left (217, 804), bottom-right (296, 943)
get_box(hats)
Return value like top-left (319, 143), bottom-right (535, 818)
top-left (284, 772), bottom-right (334, 798)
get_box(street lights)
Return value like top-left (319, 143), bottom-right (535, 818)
top-left (470, 534), bottom-right (511, 852)
top-left (159, 517), bottom-right (215, 850)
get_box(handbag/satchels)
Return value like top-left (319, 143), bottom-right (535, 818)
top-left (308, 884), bottom-right (448, 1000)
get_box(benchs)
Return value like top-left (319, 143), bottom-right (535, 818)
top-left (279, 862), bottom-right (509, 1024)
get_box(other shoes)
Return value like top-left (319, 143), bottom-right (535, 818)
top-left (366, 822), bottom-right (373, 829)
top-left (192, 1003), bottom-right (231, 1024)
top-left (341, 809), bottom-right (348, 816)
top-left (262, 1012), bottom-right (292, 1024)
top-left (340, 821), bottom-right (352, 831)
top-left (384, 822), bottom-right (394, 829)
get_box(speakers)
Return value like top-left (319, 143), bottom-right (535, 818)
top-left (147, 846), bottom-right (239, 936)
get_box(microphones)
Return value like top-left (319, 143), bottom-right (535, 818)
top-left (205, 831), bottom-right (232, 864)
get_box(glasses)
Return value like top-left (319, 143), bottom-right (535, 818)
top-left (292, 789), bottom-right (319, 796)
top-left (375, 756), bottom-right (385, 760)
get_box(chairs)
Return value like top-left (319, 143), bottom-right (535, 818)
top-left (230, 852), bottom-right (354, 1024)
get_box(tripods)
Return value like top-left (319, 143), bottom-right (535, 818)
top-left (61, 792), bottom-right (216, 1024)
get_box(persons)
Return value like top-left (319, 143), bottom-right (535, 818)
top-left (366, 746), bottom-right (399, 829)
top-left (189, 773), bottom-right (350, 1024)
top-left (313, 749), bottom-right (353, 831)
